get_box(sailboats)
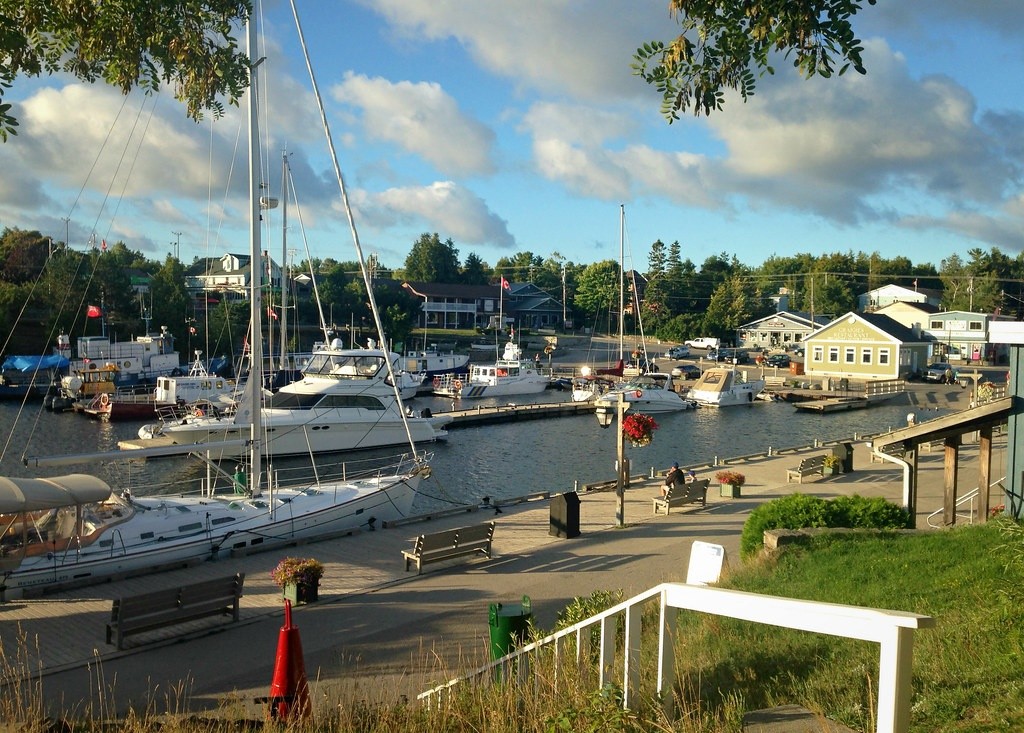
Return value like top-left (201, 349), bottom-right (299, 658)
top-left (571, 205), bottom-right (659, 402)
top-left (2, 0), bottom-right (433, 604)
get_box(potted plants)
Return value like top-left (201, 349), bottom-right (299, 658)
top-left (788, 378), bottom-right (799, 388)
top-left (504, 326), bottom-right (511, 336)
top-left (484, 325), bottom-right (496, 335)
top-left (476, 326), bottom-right (482, 334)
top-left (814, 383), bottom-right (821, 390)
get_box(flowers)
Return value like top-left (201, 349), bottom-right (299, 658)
top-left (716, 470), bottom-right (745, 487)
top-left (824, 455), bottom-right (841, 469)
top-left (269, 556), bottom-right (325, 592)
top-left (622, 412), bottom-right (660, 448)
top-left (978, 381), bottom-right (997, 398)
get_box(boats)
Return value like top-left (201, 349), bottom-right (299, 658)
top-left (0, 290), bottom-right (449, 476)
top-left (395, 304), bottom-right (553, 398)
top-left (600, 364), bottom-right (766, 416)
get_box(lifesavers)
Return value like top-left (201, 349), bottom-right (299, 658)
top-left (636, 390), bottom-right (642, 398)
top-left (433, 378), bottom-right (439, 387)
top-left (100, 393), bottom-right (109, 406)
top-left (455, 380), bottom-right (461, 389)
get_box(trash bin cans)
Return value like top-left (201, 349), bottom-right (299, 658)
top-left (831, 443), bottom-right (855, 474)
top-left (840, 378), bottom-right (848, 391)
top-left (489, 594), bottom-right (533, 684)
top-left (550, 491), bottom-right (581, 538)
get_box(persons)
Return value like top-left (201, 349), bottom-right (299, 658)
top-left (685, 471), bottom-right (697, 484)
top-left (945, 367), bottom-right (960, 385)
top-left (1007, 371), bottom-right (1011, 385)
top-left (669, 347), bottom-right (673, 359)
top-left (535, 354), bottom-right (540, 364)
top-left (195, 405), bottom-right (205, 417)
top-left (660, 462), bottom-right (686, 496)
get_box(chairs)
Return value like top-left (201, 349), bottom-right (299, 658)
top-left (18, 507), bottom-right (59, 540)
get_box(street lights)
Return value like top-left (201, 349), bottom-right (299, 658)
top-left (594, 402), bottom-right (625, 527)
top-left (530, 263), bottom-right (534, 283)
top-left (561, 262), bottom-right (566, 335)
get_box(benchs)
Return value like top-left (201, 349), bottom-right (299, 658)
top-left (918, 438), bottom-right (946, 452)
top-left (651, 477), bottom-right (711, 515)
top-left (764, 376), bottom-right (786, 386)
top-left (401, 521), bottom-right (497, 575)
top-left (869, 442), bottom-right (905, 463)
top-left (978, 426), bottom-right (1001, 437)
top-left (105, 573), bottom-right (245, 650)
top-left (786, 454), bottom-right (828, 483)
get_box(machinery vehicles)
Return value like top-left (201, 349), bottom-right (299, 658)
top-left (482, 315), bottom-right (514, 336)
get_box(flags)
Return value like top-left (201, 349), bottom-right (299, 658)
top-left (501, 277), bottom-right (512, 291)
top-left (87, 305), bottom-right (102, 318)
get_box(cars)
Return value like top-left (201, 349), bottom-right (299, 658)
top-left (671, 364), bottom-right (704, 381)
top-left (767, 349), bottom-right (805, 368)
top-left (665, 346), bottom-right (689, 359)
top-left (627, 360), bottom-right (659, 373)
top-left (707, 348), bottom-right (750, 365)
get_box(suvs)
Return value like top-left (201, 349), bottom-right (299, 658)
top-left (922, 362), bottom-right (953, 384)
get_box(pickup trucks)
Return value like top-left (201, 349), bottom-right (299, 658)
top-left (684, 337), bottom-right (721, 349)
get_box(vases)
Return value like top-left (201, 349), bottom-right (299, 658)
top-left (494, 330), bottom-right (501, 336)
top-left (827, 465), bottom-right (839, 475)
top-left (283, 580), bottom-right (319, 606)
top-left (720, 483), bottom-right (741, 499)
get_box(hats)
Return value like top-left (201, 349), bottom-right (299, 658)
top-left (670, 462), bottom-right (679, 467)
top-left (688, 471), bottom-right (695, 475)
